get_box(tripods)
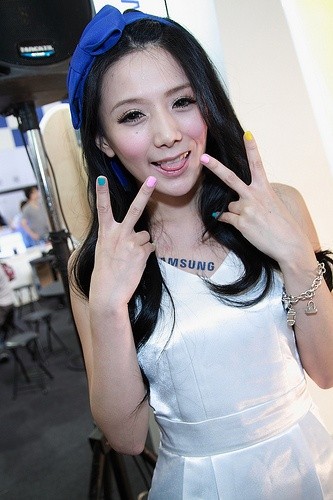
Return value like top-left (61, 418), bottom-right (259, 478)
top-left (13, 102), bottom-right (160, 500)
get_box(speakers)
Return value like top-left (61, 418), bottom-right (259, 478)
top-left (0, 0), bottom-right (97, 116)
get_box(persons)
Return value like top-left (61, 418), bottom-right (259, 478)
top-left (21, 185), bottom-right (51, 246)
top-left (17, 201), bottom-right (37, 248)
top-left (65, 4), bottom-right (333, 500)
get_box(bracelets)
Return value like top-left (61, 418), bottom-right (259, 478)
top-left (281, 259), bottom-right (327, 327)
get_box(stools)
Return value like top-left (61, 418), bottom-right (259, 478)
top-left (0, 284), bottom-right (67, 401)
